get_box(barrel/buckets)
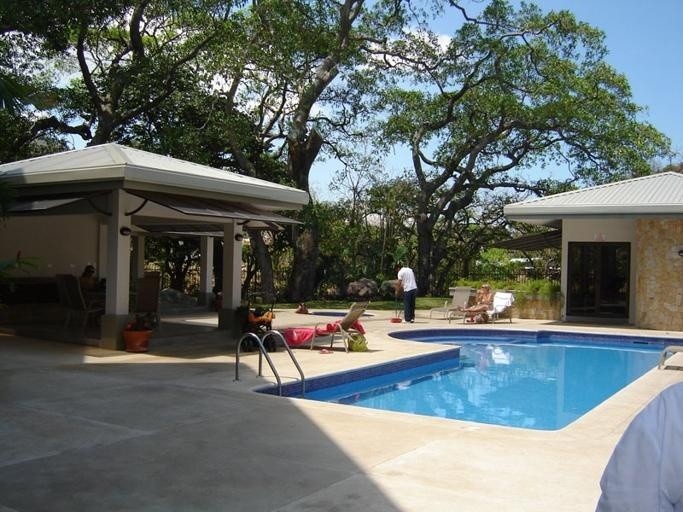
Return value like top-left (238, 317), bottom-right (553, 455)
top-left (123, 330), bottom-right (154, 353)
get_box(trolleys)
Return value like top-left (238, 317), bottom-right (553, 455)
top-left (247, 293), bottom-right (275, 331)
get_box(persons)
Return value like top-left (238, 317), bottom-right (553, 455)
top-left (460, 284), bottom-right (492, 312)
top-left (394, 265), bottom-right (417, 323)
top-left (79, 265), bottom-right (97, 290)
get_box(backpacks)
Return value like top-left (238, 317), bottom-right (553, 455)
top-left (348, 333), bottom-right (368, 352)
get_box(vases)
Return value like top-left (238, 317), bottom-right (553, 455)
top-left (122, 329), bottom-right (152, 352)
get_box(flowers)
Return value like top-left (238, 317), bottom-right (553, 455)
top-left (125, 312), bottom-right (159, 330)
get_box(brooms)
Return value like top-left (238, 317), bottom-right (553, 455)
top-left (392, 292), bottom-right (402, 322)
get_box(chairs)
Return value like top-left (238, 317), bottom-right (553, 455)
top-left (281, 302), bottom-right (371, 354)
top-left (429, 286), bottom-right (515, 325)
top-left (55, 272), bottom-right (104, 335)
